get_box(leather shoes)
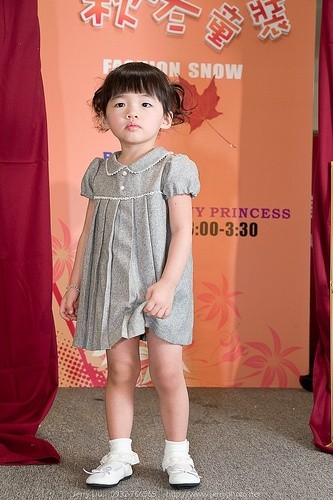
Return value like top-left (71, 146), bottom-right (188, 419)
top-left (165, 463), bottom-right (201, 488)
top-left (85, 459), bottom-right (133, 486)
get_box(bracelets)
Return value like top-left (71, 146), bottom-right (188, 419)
top-left (65, 285), bottom-right (80, 294)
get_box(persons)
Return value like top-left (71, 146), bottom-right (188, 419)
top-left (58, 59), bottom-right (206, 490)
